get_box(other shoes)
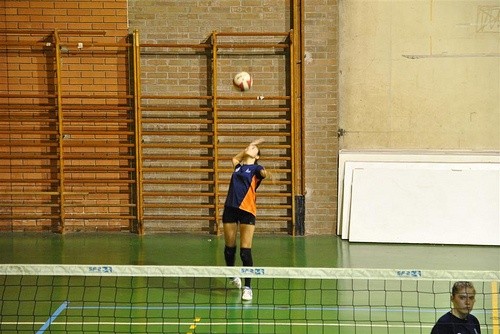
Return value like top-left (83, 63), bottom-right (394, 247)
top-left (241, 285), bottom-right (253, 300)
top-left (232, 277), bottom-right (242, 290)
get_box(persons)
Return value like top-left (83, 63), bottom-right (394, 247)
top-left (430, 281), bottom-right (482, 334)
top-left (220, 138), bottom-right (267, 301)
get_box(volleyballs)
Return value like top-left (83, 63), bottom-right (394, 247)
top-left (234, 70), bottom-right (253, 92)
